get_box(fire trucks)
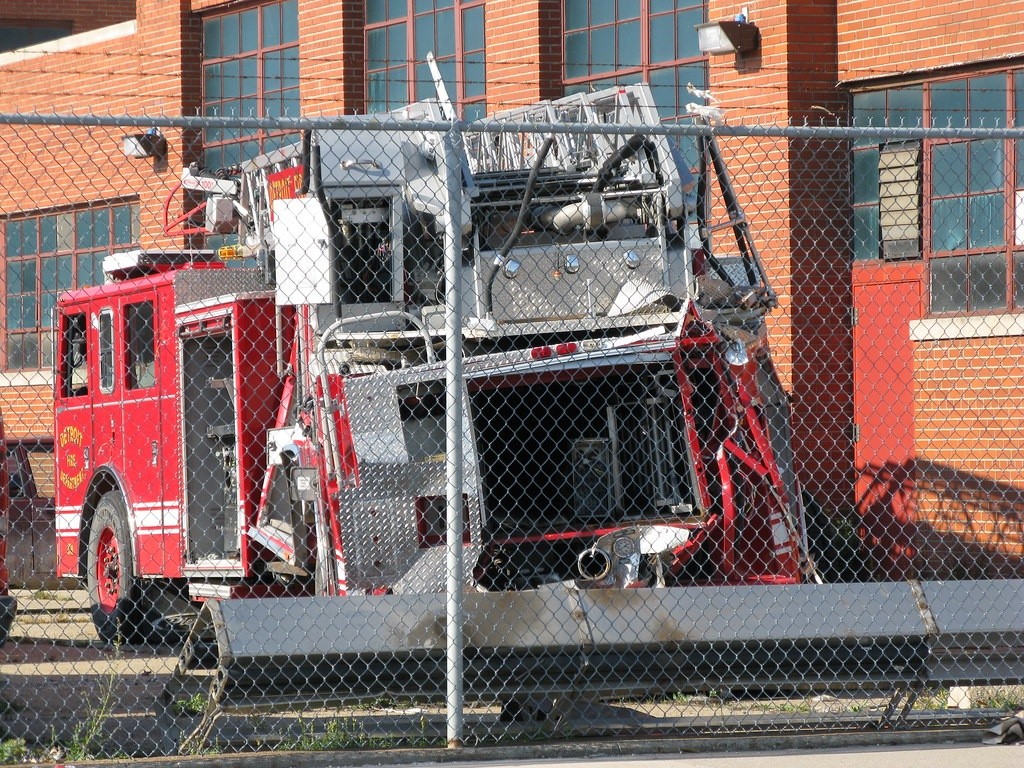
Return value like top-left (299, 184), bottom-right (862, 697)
top-left (52, 54), bottom-right (813, 645)
